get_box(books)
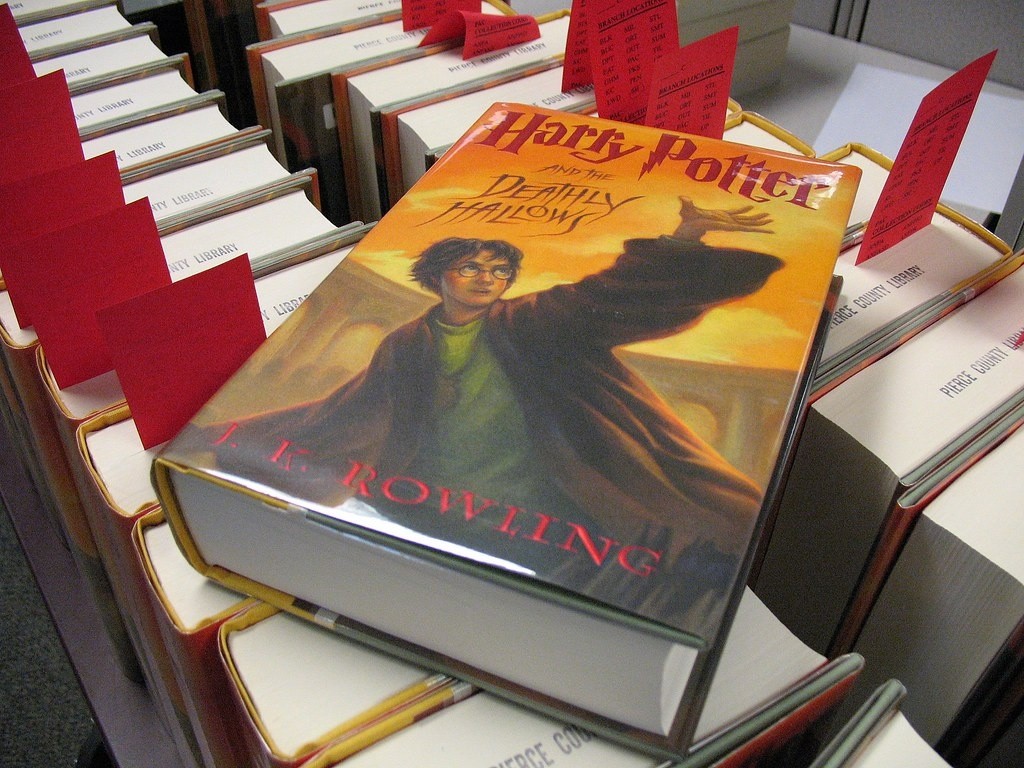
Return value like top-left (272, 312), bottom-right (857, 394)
top-left (0, 0), bottom-right (1024, 768)
top-left (151, 102), bottom-right (863, 762)
